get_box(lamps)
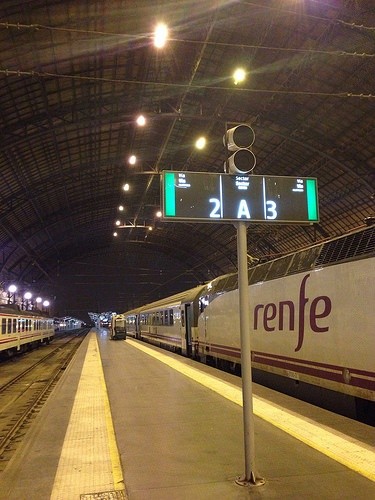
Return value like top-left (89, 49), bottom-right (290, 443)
top-left (222, 124), bottom-right (258, 176)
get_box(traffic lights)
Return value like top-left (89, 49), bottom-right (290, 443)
top-left (222, 120), bottom-right (258, 177)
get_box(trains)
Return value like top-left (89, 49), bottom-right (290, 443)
top-left (115, 218), bottom-right (374, 428)
top-left (0, 304), bottom-right (56, 362)
